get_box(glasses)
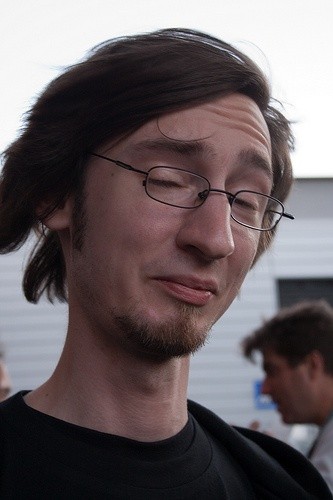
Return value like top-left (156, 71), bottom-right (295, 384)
top-left (83, 150), bottom-right (296, 232)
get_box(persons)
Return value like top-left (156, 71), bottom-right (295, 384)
top-left (243, 299), bottom-right (333, 493)
top-left (0, 27), bottom-right (333, 500)
